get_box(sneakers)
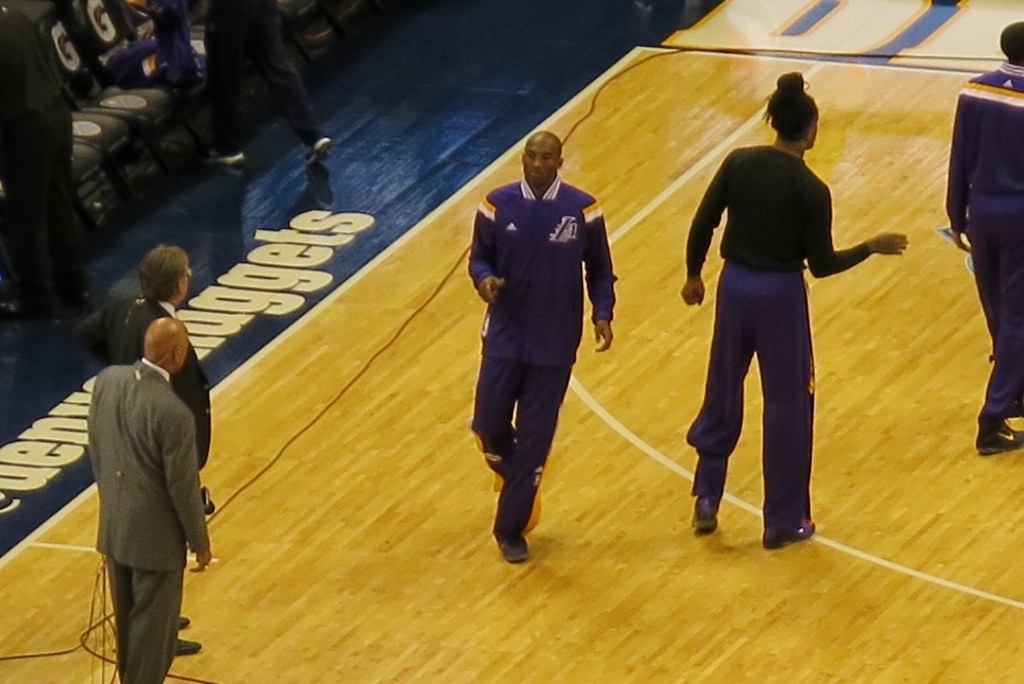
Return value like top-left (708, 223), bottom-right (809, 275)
top-left (304, 135), bottom-right (332, 164)
top-left (976, 421), bottom-right (1024, 453)
top-left (198, 145), bottom-right (244, 164)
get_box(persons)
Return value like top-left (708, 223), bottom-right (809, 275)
top-left (77, 247), bottom-right (211, 656)
top-left (0, 8), bottom-right (75, 315)
top-left (141, 0), bottom-right (332, 166)
top-left (88, 318), bottom-right (211, 684)
top-left (470, 131), bottom-right (617, 562)
top-left (680, 71), bottom-right (907, 549)
top-left (946, 22), bottom-right (1024, 453)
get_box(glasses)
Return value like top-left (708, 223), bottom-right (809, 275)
top-left (182, 269), bottom-right (192, 278)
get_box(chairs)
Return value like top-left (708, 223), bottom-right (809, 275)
top-left (25, 0), bottom-right (371, 238)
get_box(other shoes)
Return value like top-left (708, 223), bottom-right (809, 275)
top-left (763, 523), bottom-right (810, 549)
top-left (495, 533), bottom-right (529, 561)
top-left (693, 498), bottom-right (718, 532)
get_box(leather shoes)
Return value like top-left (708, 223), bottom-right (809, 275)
top-left (174, 639), bottom-right (202, 655)
top-left (178, 618), bottom-right (189, 628)
